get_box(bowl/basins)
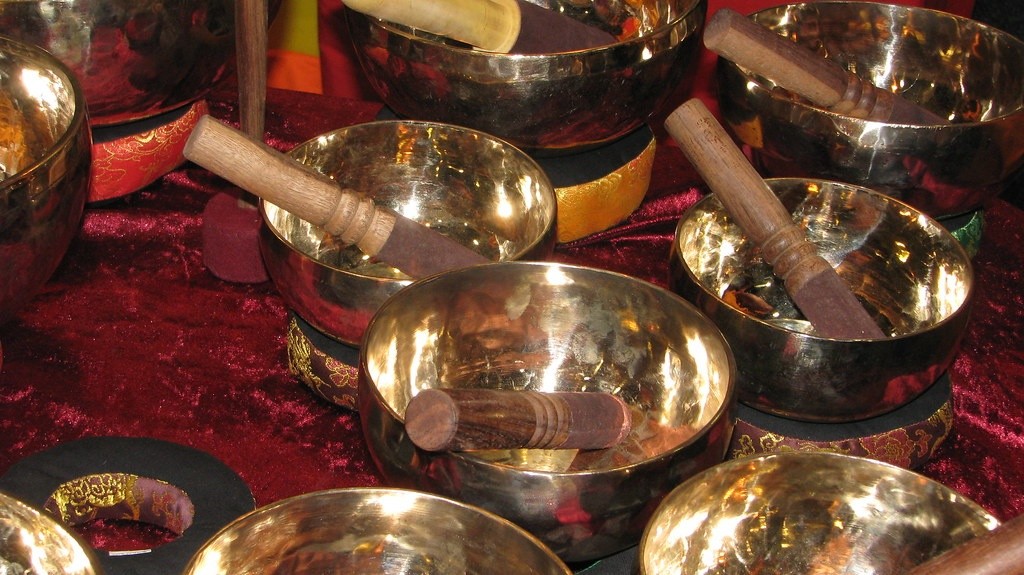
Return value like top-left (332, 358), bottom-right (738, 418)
top-left (339, 0), bottom-right (709, 158)
top-left (259, 120), bottom-right (558, 348)
top-left (0, 2), bottom-right (276, 127)
top-left (360, 262), bottom-right (739, 568)
top-left (674, 177), bottom-right (975, 421)
top-left (721, 2), bottom-right (1024, 218)
top-left (0, 38), bottom-right (94, 332)
top-left (183, 489), bottom-right (575, 575)
top-left (0, 493), bottom-right (106, 575)
top-left (638, 450), bottom-right (1004, 575)
